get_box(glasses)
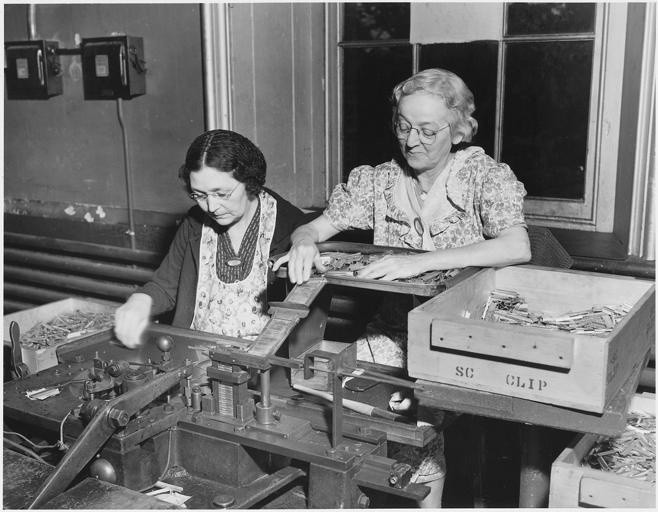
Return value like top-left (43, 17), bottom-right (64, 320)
top-left (187, 183), bottom-right (240, 202)
top-left (392, 121), bottom-right (448, 145)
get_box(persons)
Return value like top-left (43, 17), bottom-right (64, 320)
top-left (272, 69), bottom-right (532, 285)
top-left (113, 129), bottom-right (309, 349)
